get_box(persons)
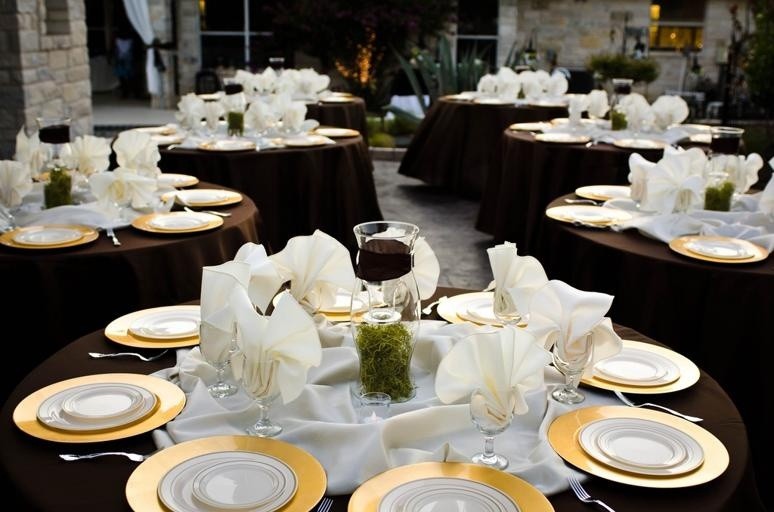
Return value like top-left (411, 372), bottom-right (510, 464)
top-left (601, 29), bottom-right (620, 55)
top-left (630, 36), bottom-right (645, 58)
top-left (114, 30), bottom-right (138, 99)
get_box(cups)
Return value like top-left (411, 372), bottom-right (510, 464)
top-left (357, 393), bottom-right (391, 423)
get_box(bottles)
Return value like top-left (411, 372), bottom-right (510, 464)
top-left (347, 220), bottom-right (421, 404)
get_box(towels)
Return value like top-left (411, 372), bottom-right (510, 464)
top-left (229, 66), bottom-right (329, 102)
top-left (660, 141), bottom-right (701, 170)
top-left (14, 124), bottom-right (41, 167)
top-left (113, 127), bottom-right (161, 167)
top-left (280, 228), bottom-right (354, 316)
top-left (487, 241), bottom-right (550, 322)
top-left (628, 153), bottom-right (657, 204)
top-left (237, 242), bottom-right (281, 310)
top-left (199, 259), bottom-right (249, 336)
top-left (75, 134), bottom-right (112, 160)
top-left (568, 87), bottom-right (690, 134)
top-left (234, 291), bottom-right (323, 405)
top-left (759, 154), bottom-right (774, 220)
top-left (177, 90), bottom-right (306, 140)
top-left (435, 322), bottom-right (553, 431)
top-left (356, 226), bottom-right (441, 299)
top-left (478, 68), bottom-right (568, 99)
top-left (710, 150), bottom-right (764, 186)
top-left (642, 165), bottom-right (704, 215)
top-left (0, 160), bottom-right (32, 207)
top-left (523, 280), bottom-right (623, 368)
top-left (94, 174), bottom-right (156, 209)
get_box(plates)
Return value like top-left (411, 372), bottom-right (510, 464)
top-left (580, 339), bottom-right (701, 395)
top-left (0, 92), bottom-right (769, 264)
top-left (103, 306), bottom-right (198, 350)
top-left (432, 292), bottom-right (526, 328)
top-left (345, 460), bottom-right (554, 512)
top-left (547, 405), bottom-right (730, 490)
top-left (123, 435), bottom-right (329, 512)
top-left (11, 373), bottom-right (188, 443)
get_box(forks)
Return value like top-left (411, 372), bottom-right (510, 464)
top-left (566, 474), bottom-right (614, 510)
top-left (61, 446), bottom-right (165, 463)
top-left (611, 389), bottom-right (706, 424)
top-left (88, 348), bottom-right (168, 362)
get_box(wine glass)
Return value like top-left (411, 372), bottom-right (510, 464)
top-left (550, 337), bottom-right (591, 405)
top-left (469, 390), bottom-right (516, 470)
top-left (239, 356), bottom-right (285, 439)
top-left (200, 319), bottom-right (238, 398)
top-left (1, 81), bottom-right (748, 223)
